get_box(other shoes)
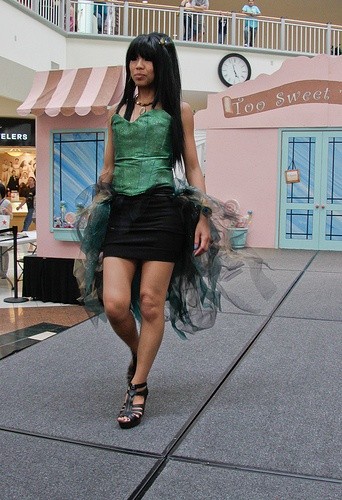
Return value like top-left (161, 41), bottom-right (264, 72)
top-left (244, 44), bottom-right (248, 47)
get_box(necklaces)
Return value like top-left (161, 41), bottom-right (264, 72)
top-left (135, 94), bottom-right (157, 115)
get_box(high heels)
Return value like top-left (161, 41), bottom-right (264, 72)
top-left (118, 381), bottom-right (148, 428)
top-left (127, 352), bottom-right (137, 385)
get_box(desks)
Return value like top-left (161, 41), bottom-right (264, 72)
top-left (0, 231), bottom-right (37, 291)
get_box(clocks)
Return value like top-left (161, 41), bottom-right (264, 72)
top-left (218, 53), bottom-right (251, 87)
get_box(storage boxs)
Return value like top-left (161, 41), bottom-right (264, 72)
top-left (227, 227), bottom-right (249, 249)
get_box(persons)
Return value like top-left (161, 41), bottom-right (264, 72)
top-left (68, 0), bottom-right (108, 37)
top-left (72, 34), bottom-right (276, 427)
top-left (0, 156), bottom-right (36, 189)
top-left (184, 0), bottom-right (263, 47)
top-left (0, 170), bottom-right (36, 279)
